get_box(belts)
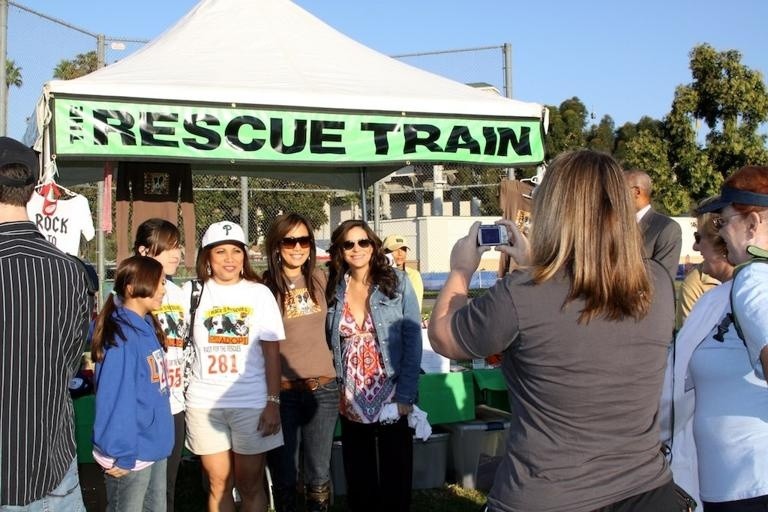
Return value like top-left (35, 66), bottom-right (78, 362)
top-left (281, 377), bottom-right (336, 390)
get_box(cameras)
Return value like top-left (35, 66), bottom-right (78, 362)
top-left (478, 224), bottom-right (508, 246)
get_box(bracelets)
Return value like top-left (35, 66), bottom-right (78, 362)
top-left (267, 393), bottom-right (281, 404)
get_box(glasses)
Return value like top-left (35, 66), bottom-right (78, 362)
top-left (339, 239), bottom-right (372, 248)
top-left (279, 237), bottom-right (314, 249)
top-left (709, 214), bottom-right (763, 227)
top-left (694, 231), bottom-right (714, 244)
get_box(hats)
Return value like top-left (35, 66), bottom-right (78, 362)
top-left (382, 234), bottom-right (412, 253)
top-left (0, 134), bottom-right (41, 189)
top-left (695, 183), bottom-right (768, 217)
top-left (199, 220), bottom-right (252, 250)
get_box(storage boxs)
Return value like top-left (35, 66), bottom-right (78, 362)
top-left (412, 431), bottom-right (451, 491)
top-left (445, 405), bottom-right (513, 490)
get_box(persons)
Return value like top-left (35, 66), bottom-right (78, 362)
top-left (381, 232), bottom-right (424, 314)
top-left (657, 164), bottom-right (767, 511)
top-left (1, 134), bottom-right (97, 512)
top-left (261, 211), bottom-right (346, 512)
top-left (90, 254), bottom-right (176, 512)
top-left (676, 261), bottom-right (724, 325)
top-left (658, 196), bottom-right (734, 510)
top-left (131, 216), bottom-right (189, 512)
top-left (425, 148), bottom-right (699, 512)
top-left (181, 220), bottom-right (287, 511)
top-left (624, 170), bottom-right (682, 281)
top-left (326, 215), bottom-right (424, 511)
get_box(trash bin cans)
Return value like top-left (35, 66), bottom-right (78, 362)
top-left (69, 376), bottom-right (97, 465)
